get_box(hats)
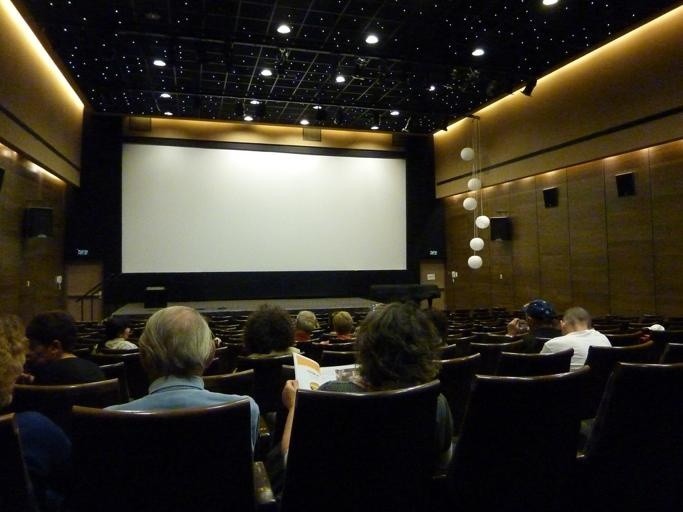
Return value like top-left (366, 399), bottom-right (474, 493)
top-left (642, 324), bottom-right (665, 331)
top-left (522, 300), bottom-right (552, 318)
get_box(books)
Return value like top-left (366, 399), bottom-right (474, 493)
top-left (290, 351), bottom-right (363, 391)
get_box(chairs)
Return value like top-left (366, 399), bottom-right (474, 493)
top-left (0, 306), bottom-right (683, 512)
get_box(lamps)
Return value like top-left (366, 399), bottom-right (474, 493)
top-left (458, 114), bottom-right (492, 271)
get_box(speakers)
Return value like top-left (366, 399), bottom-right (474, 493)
top-left (24, 207), bottom-right (53, 239)
top-left (490, 216), bottom-right (512, 241)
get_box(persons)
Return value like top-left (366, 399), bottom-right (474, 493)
top-left (281, 302), bottom-right (454, 469)
top-left (103, 307), bottom-right (259, 471)
top-left (506, 298), bottom-right (557, 340)
top-left (0, 312), bottom-right (74, 505)
top-left (540, 307), bottom-right (613, 373)
top-left (640, 324), bottom-right (666, 343)
